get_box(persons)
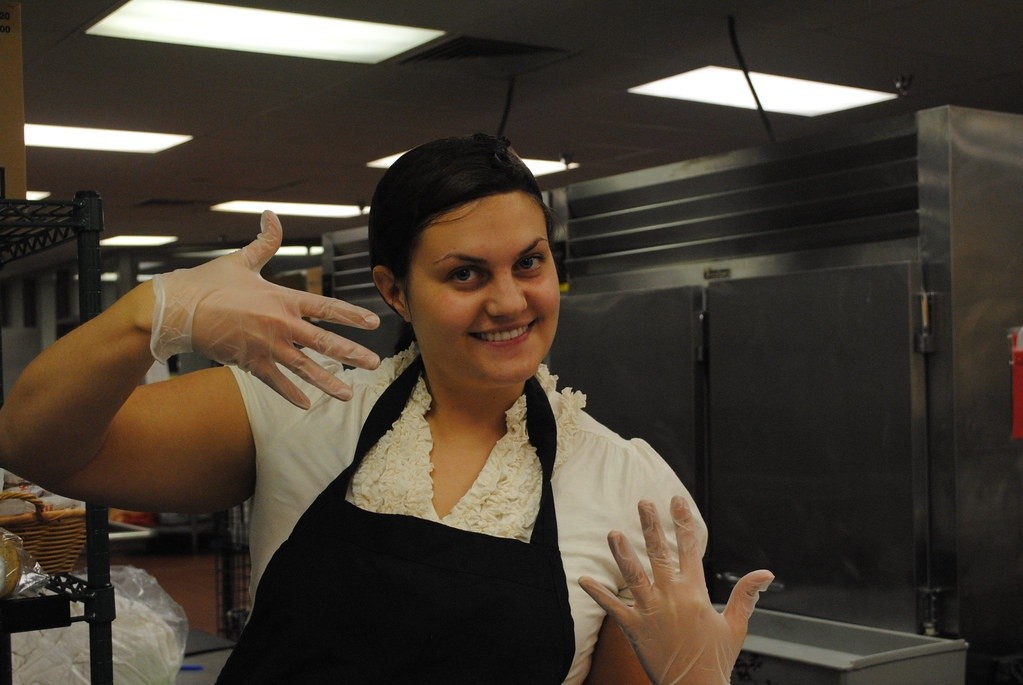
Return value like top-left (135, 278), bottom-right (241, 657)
top-left (0, 134), bottom-right (776, 685)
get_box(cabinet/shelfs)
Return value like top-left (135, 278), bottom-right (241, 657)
top-left (0, 187), bottom-right (117, 685)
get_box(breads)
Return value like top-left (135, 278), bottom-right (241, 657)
top-left (0, 535), bottom-right (22, 599)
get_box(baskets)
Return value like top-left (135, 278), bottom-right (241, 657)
top-left (0, 490), bottom-right (85, 573)
